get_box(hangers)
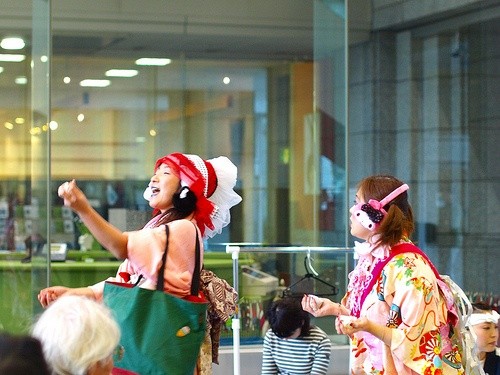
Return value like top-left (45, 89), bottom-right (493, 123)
top-left (282, 255), bottom-right (339, 299)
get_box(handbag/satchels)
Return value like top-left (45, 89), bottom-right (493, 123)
top-left (103, 221), bottom-right (208, 374)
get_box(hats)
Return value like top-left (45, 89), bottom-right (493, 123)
top-left (156, 152), bottom-right (241, 237)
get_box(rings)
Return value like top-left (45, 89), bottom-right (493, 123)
top-left (313, 308), bottom-right (317, 311)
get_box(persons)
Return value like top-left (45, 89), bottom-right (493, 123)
top-left (261, 297), bottom-right (331, 375)
top-left (299, 175), bottom-right (464, 375)
top-left (38, 153), bottom-right (241, 375)
top-left (0, 294), bottom-right (121, 375)
top-left (458, 302), bottom-right (500, 375)
